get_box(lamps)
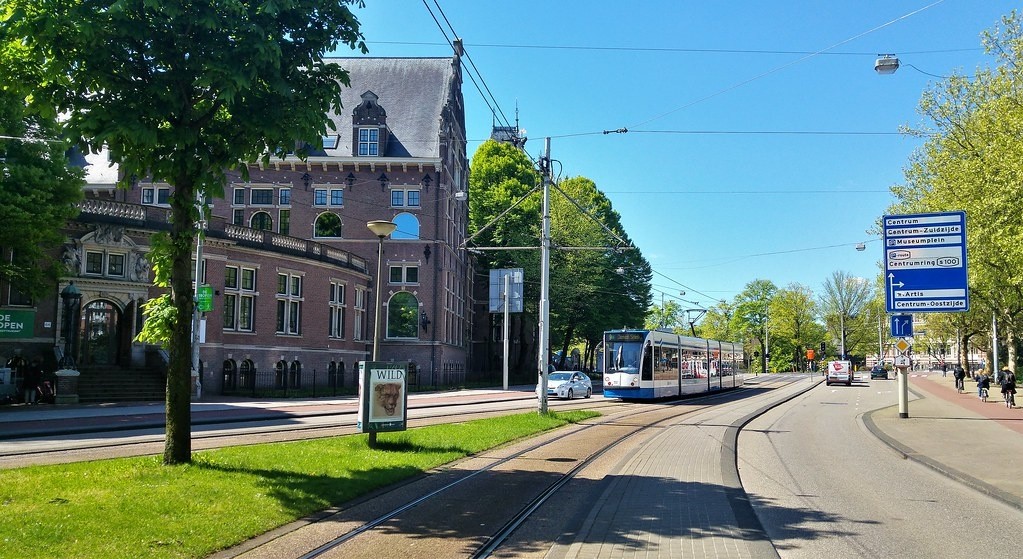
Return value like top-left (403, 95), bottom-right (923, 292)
top-left (455, 190), bottom-right (467, 200)
top-left (420, 311), bottom-right (431, 333)
top-left (875, 54), bottom-right (899, 74)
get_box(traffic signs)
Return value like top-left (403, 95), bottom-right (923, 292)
top-left (882, 209), bottom-right (971, 314)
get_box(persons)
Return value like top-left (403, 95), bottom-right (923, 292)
top-left (975, 369), bottom-right (990, 398)
top-left (24, 364), bottom-right (40, 407)
top-left (954, 364), bottom-right (965, 390)
top-left (998, 366), bottom-right (1017, 406)
top-left (940, 362), bottom-right (947, 377)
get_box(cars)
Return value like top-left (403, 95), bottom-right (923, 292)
top-left (535, 370), bottom-right (593, 400)
top-left (870, 365), bottom-right (889, 380)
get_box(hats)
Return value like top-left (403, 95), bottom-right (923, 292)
top-left (1002, 366), bottom-right (1009, 371)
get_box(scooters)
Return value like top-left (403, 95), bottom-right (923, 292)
top-left (1004, 389), bottom-right (1013, 409)
top-left (957, 378), bottom-right (963, 394)
top-left (981, 388), bottom-right (989, 403)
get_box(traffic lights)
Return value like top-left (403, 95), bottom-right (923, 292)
top-left (820, 342), bottom-right (826, 351)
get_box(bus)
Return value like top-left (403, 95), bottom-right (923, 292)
top-left (593, 307), bottom-right (745, 403)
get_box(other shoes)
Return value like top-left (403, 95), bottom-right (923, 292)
top-left (1011, 402), bottom-right (1015, 406)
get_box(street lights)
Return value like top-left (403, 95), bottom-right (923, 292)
top-left (365, 219), bottom-right (398, 450)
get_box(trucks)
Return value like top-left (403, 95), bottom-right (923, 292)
top-left (826, 359), bottom-right (854, 386)
top-left (681, 356), bottom-right (722, 378)
